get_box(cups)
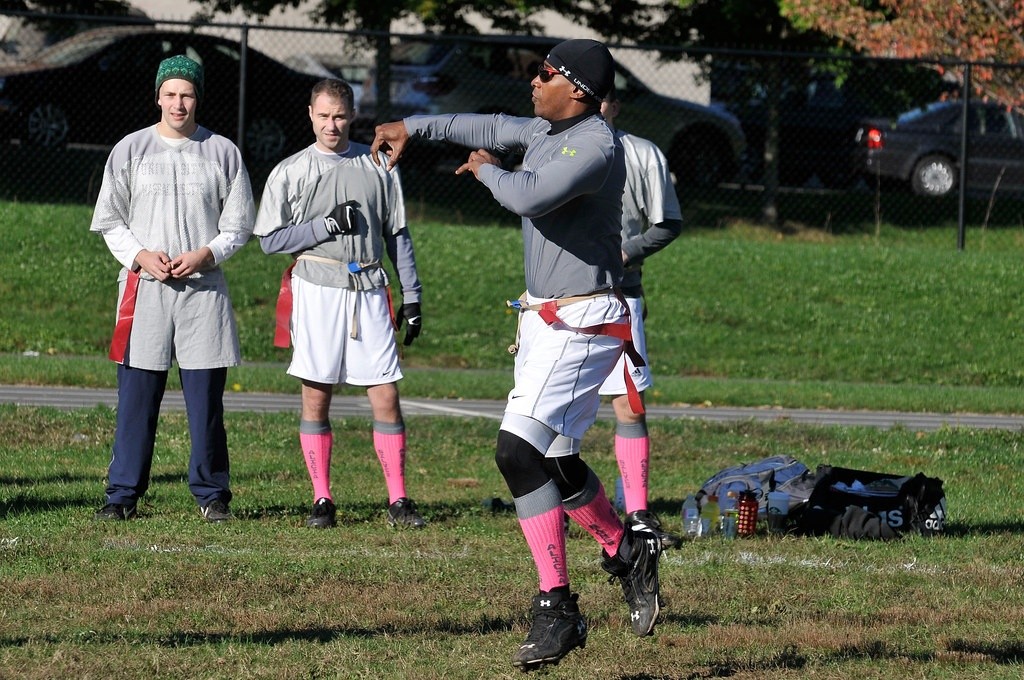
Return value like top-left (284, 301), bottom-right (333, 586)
top-left (767, 492), bottom-right (791, 515)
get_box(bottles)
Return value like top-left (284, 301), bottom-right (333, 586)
top-left (682, 493), bottom-right (698, 543)
top-left (701, 495), bottom-right (721, 538)
top-left (614, 475), bottom-right (625, 514)
top-left (723, 488), bottom-right (764, 540)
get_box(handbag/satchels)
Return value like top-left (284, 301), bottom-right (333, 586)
top-left (696, 454), bottom-right (816, 516)
top-left (781, 464), bottom-right (948, 540)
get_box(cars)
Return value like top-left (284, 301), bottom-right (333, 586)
top-left (0, 27), bottom-right (368, 179)
top-left (846, 100), bottom-right (1024, 201)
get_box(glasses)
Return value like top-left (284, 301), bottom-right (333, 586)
top-left (537, 64), bottom-right (562, 82)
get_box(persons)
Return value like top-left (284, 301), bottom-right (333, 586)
top-left (556, 86), bottom-right (685, 548)
top-left (89, 52), bottom-right (257, 526)
top-left (368, 39), bottom-right (665, 669)
top-left (250, 78), bottom-right (425, 530)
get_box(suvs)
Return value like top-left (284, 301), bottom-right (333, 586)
top-left (351, 36), bottom-right (749, 203)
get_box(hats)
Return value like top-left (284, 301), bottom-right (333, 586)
top-left (155, 55), bottom-right (204, 111)
top-left (546, 39), bottom-right (615, 103)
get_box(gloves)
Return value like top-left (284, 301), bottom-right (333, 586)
top-left (395, 303), bottom-right (421, 345)
top-left (323, 199), bottom-right (358, 234)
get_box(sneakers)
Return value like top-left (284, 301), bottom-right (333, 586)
top-left (602, 527), bottom-right (662, 637)
top-left (623, 510), bottom-right (678, 545)
top-left (386, 496), bottom-right (425, 528)
top-left (513, 591), bottom-right (587, 671)
top-left (94, 501), bottom-right (138, 520)
top-left (307, 499), bottom-right (336, 527)
top-left (199, 499), bottom-right (230, 520)
